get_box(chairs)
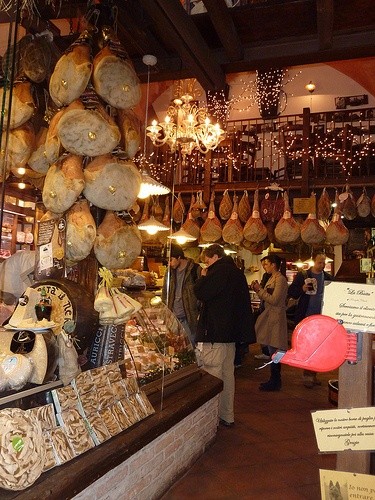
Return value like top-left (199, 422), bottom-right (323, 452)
top-left (211, 124), bottom-right (375, 182)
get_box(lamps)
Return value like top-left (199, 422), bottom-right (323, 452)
top-left (137, 55), bottom-right (170, 199)
top-left (305, 81), bottom-right (315, 93)
top-left (167, 213), bottom-right (197, 245)
top-left (138, 216), bottom-right (170, 235)
top-left (292, 247), bottom-right (333, 268)
top-left (222, 244), bottom-right (237, 255)
top-left (145, 78), bottom-right (225, 155)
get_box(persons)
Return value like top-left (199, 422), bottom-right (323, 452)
top-left (161, 242), bottom-right (203, 344)
top-left (249, 254), bottom-right (289, 393)
top-left (193, 244), bottom-right (257, 426)
top-left (288, 251), bottom-right (335, 388)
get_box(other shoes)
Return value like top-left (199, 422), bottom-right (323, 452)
top-left (234, 364), bottom-right (243, 368)
top-left (254, 353), bottom-right (271, 359)
top-left (314, 379), bottom-right (322, 385)
top-left (259, 380), bottom-right (282, 392)
top-left (221, 419), bottom-right (234, 426)
top-left (304, 379), bottom-right (312, 388)
